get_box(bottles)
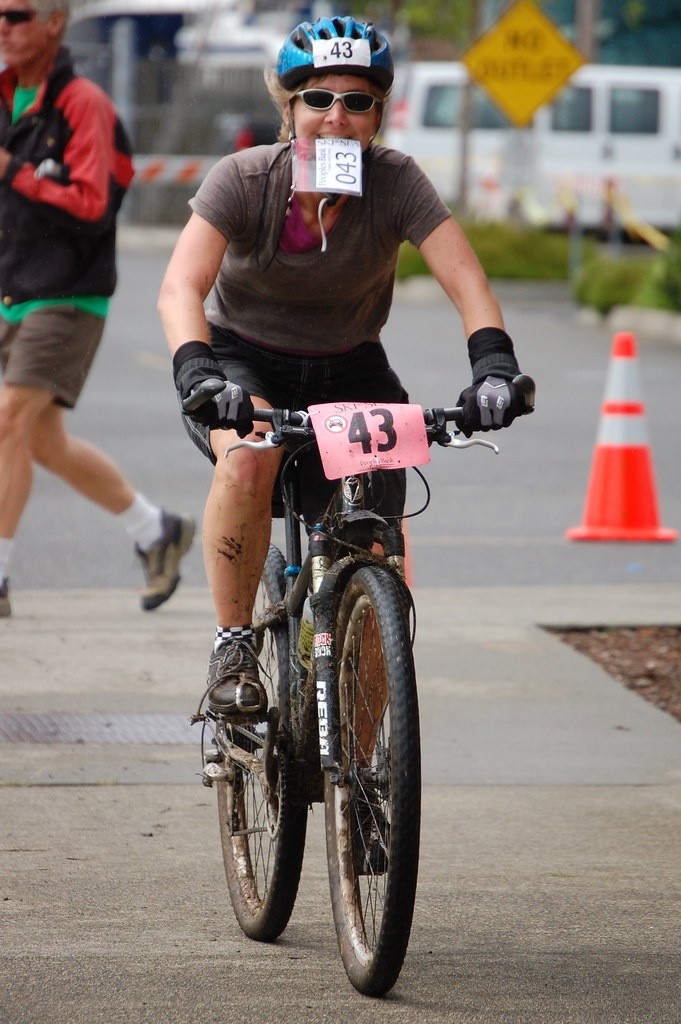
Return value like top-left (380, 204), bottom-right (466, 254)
top-left (298, 583), bottom-right (322, 670)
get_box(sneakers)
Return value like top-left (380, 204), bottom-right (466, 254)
top-left (132, 507), bottom-right (196, 612)
top-left (206, 635), bottom-right (273, 717)
top-left (347, 786), bottom-right (391, 876)
top-left (0, 576), bottom-right (11, 618)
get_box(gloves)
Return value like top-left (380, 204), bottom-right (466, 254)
top-left (455, 376), bottom-right (525, 438)
top-left (188, 379), bottom-right (256, 440)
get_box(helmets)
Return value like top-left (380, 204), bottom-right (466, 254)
top-left (277, 16), bottom-right (395, 91)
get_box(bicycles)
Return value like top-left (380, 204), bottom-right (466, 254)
top-left (189, 364), bottom-right (541, 1001)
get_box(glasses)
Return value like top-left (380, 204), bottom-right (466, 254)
top-left (288, 86), bottom-right (383, 115)
top-left (0, 9), bottom-right (33, 24)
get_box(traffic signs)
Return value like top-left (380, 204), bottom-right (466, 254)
top-left (456, 0), bottom-right (585, 130)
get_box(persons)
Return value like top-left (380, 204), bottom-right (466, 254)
top-left (154, 18), bottom-right (540, 878)
top-left (1, 2), bottom-right (196, 623)
